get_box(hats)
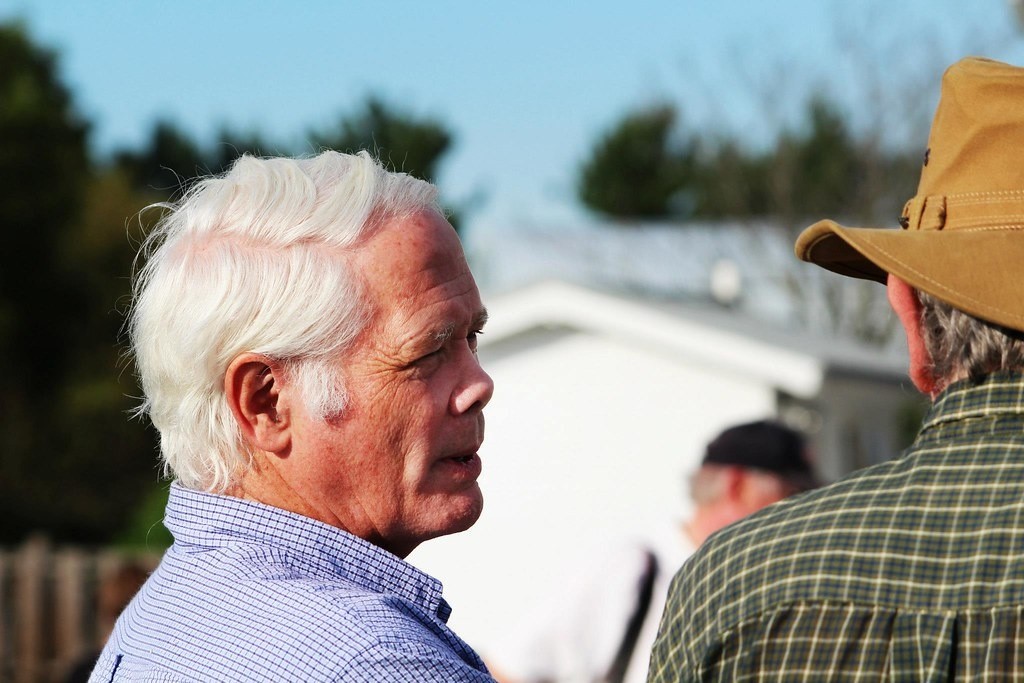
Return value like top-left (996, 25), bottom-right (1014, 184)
top-left (794, 57), bottom-right (1024, 333)
top-left (703, 422), bottom-right (816, 484)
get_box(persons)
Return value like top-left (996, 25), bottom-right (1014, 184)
top-left (86, 148), bottom-right (500, 682)
top-left (597, 420), bottom-right (820, 683)
top-left (646, 57), bottom-right (1024, 683)
